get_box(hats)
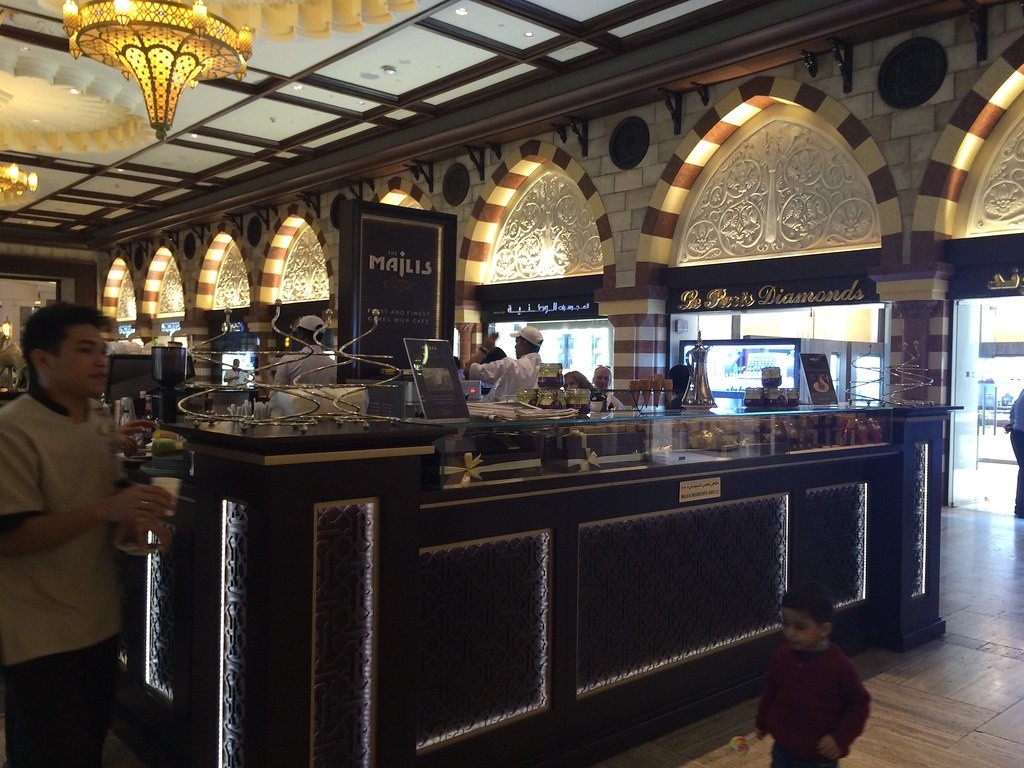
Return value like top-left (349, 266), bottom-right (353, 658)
top-left (509, 325), bottom-right (544, 348)
top-left (297, 314), bottom-right (326, 334)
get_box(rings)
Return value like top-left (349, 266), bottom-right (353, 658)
top-left (141, 500), bottom-right (146, 509)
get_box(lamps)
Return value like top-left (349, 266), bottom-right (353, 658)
top-left (0, 163), bottom-right (38, 197)
top-left (62, 0), bottom-right (256, 141)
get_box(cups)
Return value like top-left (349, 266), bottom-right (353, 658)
top-left (785, 387), bottom-right (799, 410)
top-left (514, 388), bottom-right (537, 406)
top-left (152, 477), bottom-right (181, 517)
top-left (762, 367), bottom-right (781, 387)
top-left (567, 389), bottom-right (591, 418)
top-left (539, 391), bottom-right (567, 410)
top-left (746, 387), bottom-right (763, 408)
top-left (765, 389), bottom-right (788, 410)
top-left (537, 363), bottom-right (564, 386)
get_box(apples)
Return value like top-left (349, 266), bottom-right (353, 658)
top-left (152, 438), bottom-right (176, 458)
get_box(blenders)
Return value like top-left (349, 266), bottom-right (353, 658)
top-left (152, 346), bottom-right (185, 423)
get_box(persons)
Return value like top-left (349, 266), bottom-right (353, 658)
top-left (0, 300), bottom-right (249, 768)
top-left (1004, 389), bottom-right (1024, 518)
top-left (753, 579), bottom-right (871, 768)
top-left (452, 325), bottom-right (690, 410)
top-left (267, 314), bottom-right (337, 398)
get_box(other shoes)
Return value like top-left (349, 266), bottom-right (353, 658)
top-left (1014, 505), bottom-right (1024, 518)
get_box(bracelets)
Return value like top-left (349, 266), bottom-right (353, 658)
top-left (479, 348), bottom-right (487, 355)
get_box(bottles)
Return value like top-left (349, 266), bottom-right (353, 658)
top-left (137, 390), bottom-right (157, 443)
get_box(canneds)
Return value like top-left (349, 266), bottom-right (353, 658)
top-left (744, 366), bottom-right (799, 410)
top-left (796, 412), bottom-right (856, 447)
top-left (516, 362), bottom-right (591, 415)
